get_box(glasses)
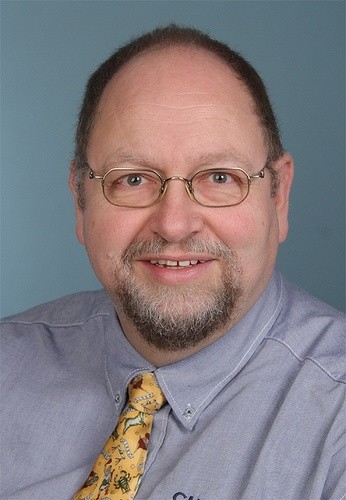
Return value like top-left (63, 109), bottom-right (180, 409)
top-left (82, 155), bottom-right (277, 208)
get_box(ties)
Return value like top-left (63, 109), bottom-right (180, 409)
top-left (72, 372), bottom-right (167, 499)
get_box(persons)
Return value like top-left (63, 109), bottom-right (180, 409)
top-left (0, 25), bottom-right (346, 498)
